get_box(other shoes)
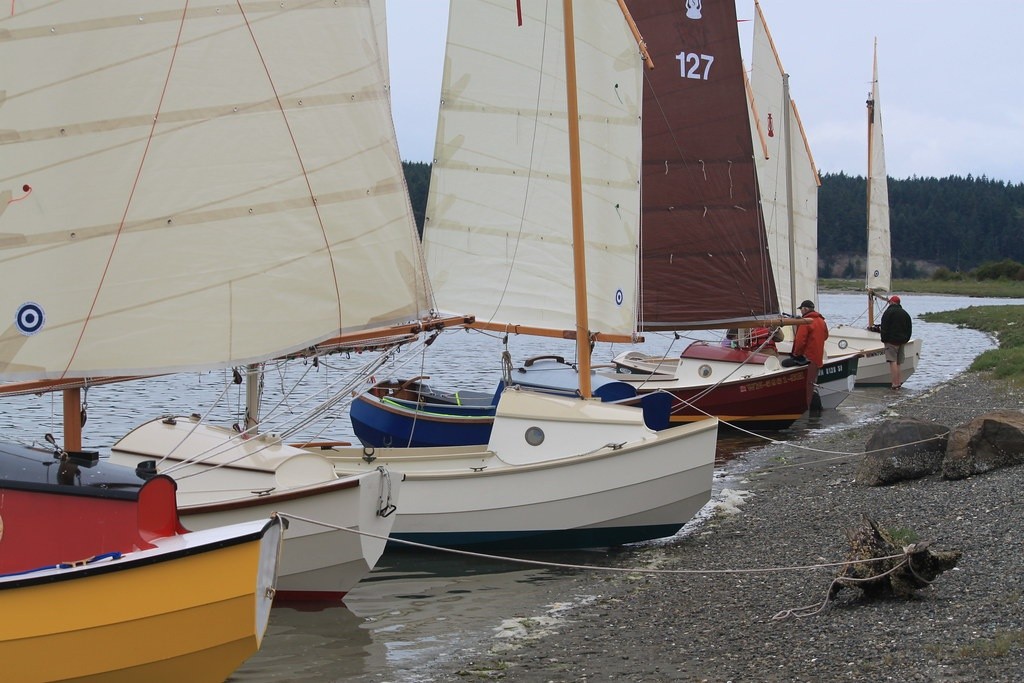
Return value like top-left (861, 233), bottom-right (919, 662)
top-left (890, 386), bottom-right (899, 389)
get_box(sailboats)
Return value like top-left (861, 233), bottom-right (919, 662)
top-left (779, 35), bottom-right (924, 390)
top-left (0, 0), bottom-right (481, 682)
top-left (108, 1), bottom-right (862, 607)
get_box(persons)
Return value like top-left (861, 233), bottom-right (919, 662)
top-left (727, 327), bottom-right (784, 357)
top-left (792, 300), bottom-right (829, 419)
top-left (881, 296), bottom-right (913, 390)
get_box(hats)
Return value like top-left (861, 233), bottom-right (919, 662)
top-left (797, 300), bottom-right (814, 309)
top-left (888, 296), bottom-right (900, 302)
